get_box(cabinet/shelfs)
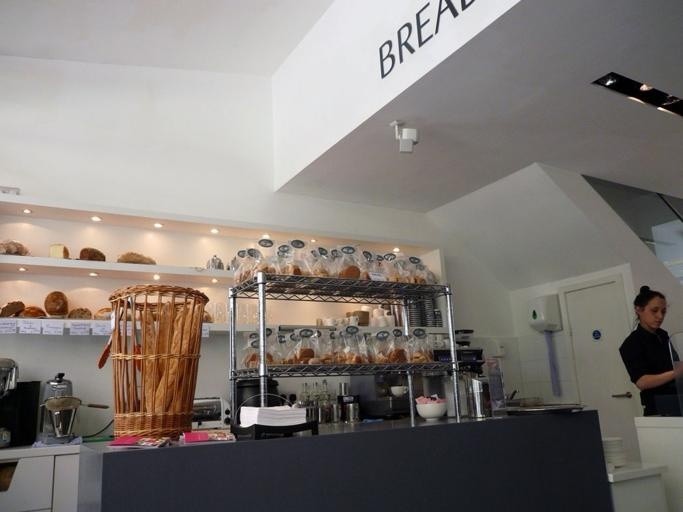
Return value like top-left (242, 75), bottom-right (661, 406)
top-left (0, 251), bottom-right (453, 337)
top-left (223, 268), bottom-right (465, 426)
top-left (0, 443), bottom-right (80, 511)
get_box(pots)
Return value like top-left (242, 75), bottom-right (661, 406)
top-left (233, 378), bottom-right (281, 426)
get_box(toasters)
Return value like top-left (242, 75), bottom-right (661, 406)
top-left (190, 396), bottom-right (231, 431)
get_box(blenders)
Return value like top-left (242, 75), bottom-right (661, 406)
top-left (0, 356), bottom-right (40, 449)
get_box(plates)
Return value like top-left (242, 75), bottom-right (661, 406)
top-left (601, 437), bottom-right (627, 468)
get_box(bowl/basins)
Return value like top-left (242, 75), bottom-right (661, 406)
top-left (414, 403), bottom-right (446, 422)
top-left (346, 306), bottom-right (396, 327)
top-left (407, 294), bottom-right (443, 327)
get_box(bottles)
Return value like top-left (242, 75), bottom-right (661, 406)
top-left (299, 380), bottom-right (330, 422)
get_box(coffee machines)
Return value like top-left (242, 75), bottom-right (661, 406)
top-left (351, 348), bottom-right (492, 417)
top-left (34, 372), bottom-right (80, 444)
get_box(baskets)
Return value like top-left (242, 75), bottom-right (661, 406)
top-left (109, 283), bottom-right (209, 440)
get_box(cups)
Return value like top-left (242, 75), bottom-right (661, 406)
top-left (426, 334), bottom-right (457, 347)
top-left (390, 384), bottom-right (410, 398)
top-left (454, 328), bottom-right (473, 345)
top-left (206, 302), bottom-right (271, 324)
top-left (323, 315), bottom-right (358, 328)
top-left (316, 319), bottom-right (321, 325)
top-left (337, 381), bottom-right (350, 396)
top-left (337, 394), bottom-right (358, 423)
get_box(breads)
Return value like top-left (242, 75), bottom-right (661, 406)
top-left (0, 238), bottom-right (157, 266)
top-left (1, 291), bottom-right (214, 323)
top-left (231, 262), bottom-right (431, 285)
top-left (242, 345), bottom-right (435, 368)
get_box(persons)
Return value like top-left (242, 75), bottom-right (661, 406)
top-left (620, 285), bottom-right (682, 417)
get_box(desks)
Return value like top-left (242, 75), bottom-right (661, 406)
top-left (633, 415), bottom-right (682, 512)
top-left (605, 459), bottom-right (673, 512)
